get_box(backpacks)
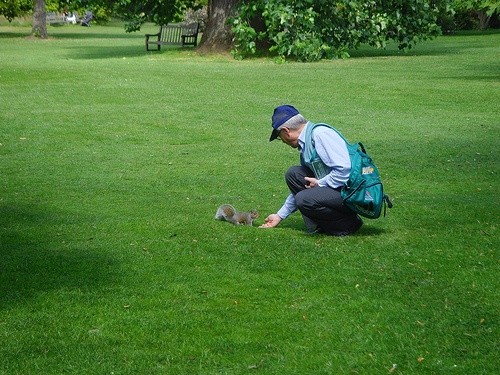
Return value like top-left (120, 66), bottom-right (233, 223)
top-left (306, 122), bottom-right (394, 219)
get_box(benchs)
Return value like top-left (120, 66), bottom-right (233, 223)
top-left (145, 23), bottom-right (200, 51)
top-left (46, 14), bottom-right (65, 25)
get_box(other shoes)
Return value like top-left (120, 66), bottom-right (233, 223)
top-left (304, 227), bottom-right (322, 235)
top-left (331, 220), bottom-right (363, 236)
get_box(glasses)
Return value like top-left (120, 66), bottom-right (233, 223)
top-left (276, 127), bottom-right (289, 140)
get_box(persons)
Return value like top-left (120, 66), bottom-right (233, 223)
top-left (258, 104), bottom-right (384, 237)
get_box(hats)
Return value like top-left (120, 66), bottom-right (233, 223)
top-left (269, 104), bottom-right (299, 142)
